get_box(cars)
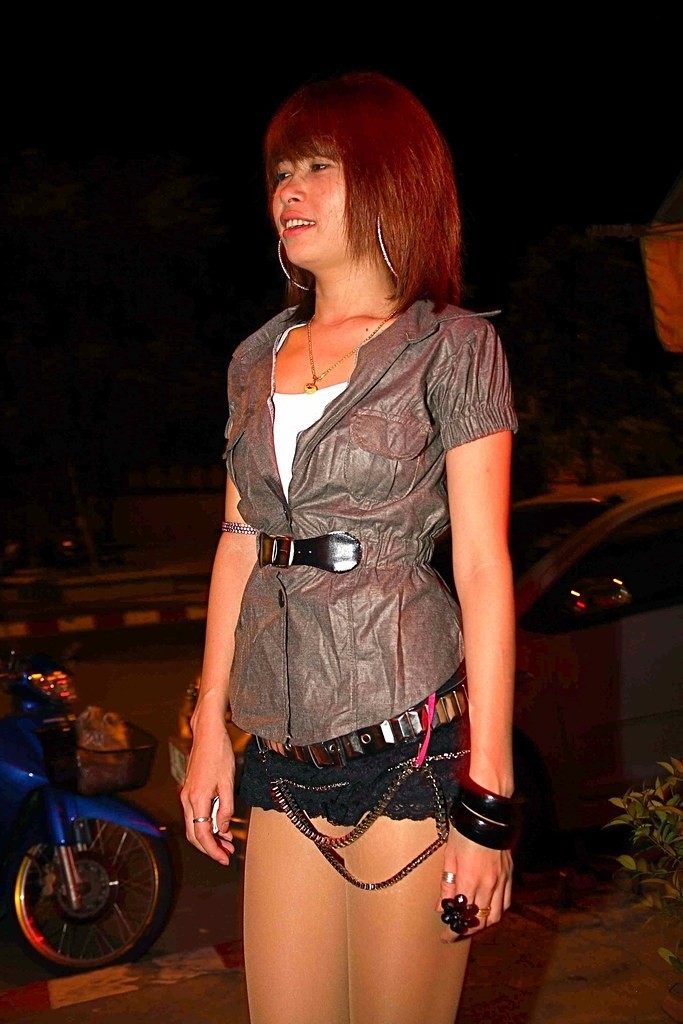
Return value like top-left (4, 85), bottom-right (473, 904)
top-left (436, 478), bottom-right (682, 857)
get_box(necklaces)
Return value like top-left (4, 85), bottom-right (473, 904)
top-left (304, 313), bottom-right (396, 394)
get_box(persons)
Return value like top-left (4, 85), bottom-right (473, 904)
top-left (561, 547), bottom-right (633, 614)
top-left (180, 74), bottom-right (524, 1024)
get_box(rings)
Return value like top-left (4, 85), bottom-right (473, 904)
top-left (193, 817), bottom-right (213, 823)
top-left (477, 908), bottom-right (491, 917)
top-left (440, 893), bottom-right (481, 936)
top-left (442, 872), bottom-right (457, 883)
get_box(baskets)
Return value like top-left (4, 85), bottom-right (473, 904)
top-left (73, 721), bottom-right (159, 794)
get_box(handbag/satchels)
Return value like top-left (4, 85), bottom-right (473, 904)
top-left (74, 707), bottom-right (135, 789)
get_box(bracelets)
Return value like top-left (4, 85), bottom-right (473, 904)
top-left (447, 800), bottom-right (514, 851)
top-left (459, 772), bottom-right (523, 827)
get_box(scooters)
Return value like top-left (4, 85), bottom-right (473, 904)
top-left (0, 656), bottom-right (180, 977)
